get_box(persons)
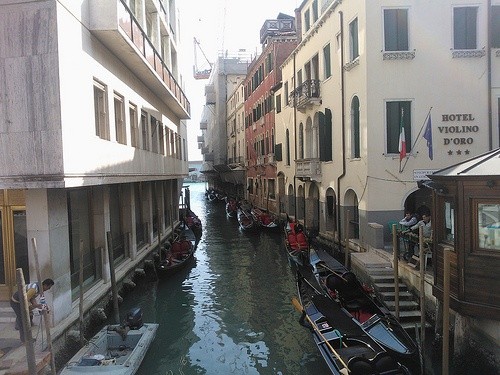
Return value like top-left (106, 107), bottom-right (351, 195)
top-left (294, 221), bottom-right (303, 234)
top-left (11, 278), bottom-right (54, 330)
top-left (410, 212), bottom-right (431, 238)
top-left (399, 211), bottom-right (418, 254)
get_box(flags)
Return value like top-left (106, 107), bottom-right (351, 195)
top-left (398, 116), bottom-right (406, 160)
top-left (424, 115), bottom-right (433, 161)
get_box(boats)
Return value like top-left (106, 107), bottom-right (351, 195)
top-left (179, 203), bottom-right (202, 235)
top-left (283, 211), bottom-right (311, 268)
top-left (56, 307), bottom-right (160, 375)
top-left (158, 220), bottom-right (196, 275)
top-left (204, 187), bottom-right (278, 231)
top-left (308, 243), bottom-right (418, 359)
top-left (294, 262), bottom-right (413, 375)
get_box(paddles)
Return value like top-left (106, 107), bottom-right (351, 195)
top-left (241, 211), bottom-right (249, 220)
top-left (321, 263), bottom-right (347, 282)
top-left (292, 298), bottom-right (351, 373)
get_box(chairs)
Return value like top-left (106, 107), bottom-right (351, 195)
top-left (289, 232), bottom-right (307, 249)
top-left (389, 221), bottom-right (409, 241)
top-left (172, 240), bottom-right (190, 253)
